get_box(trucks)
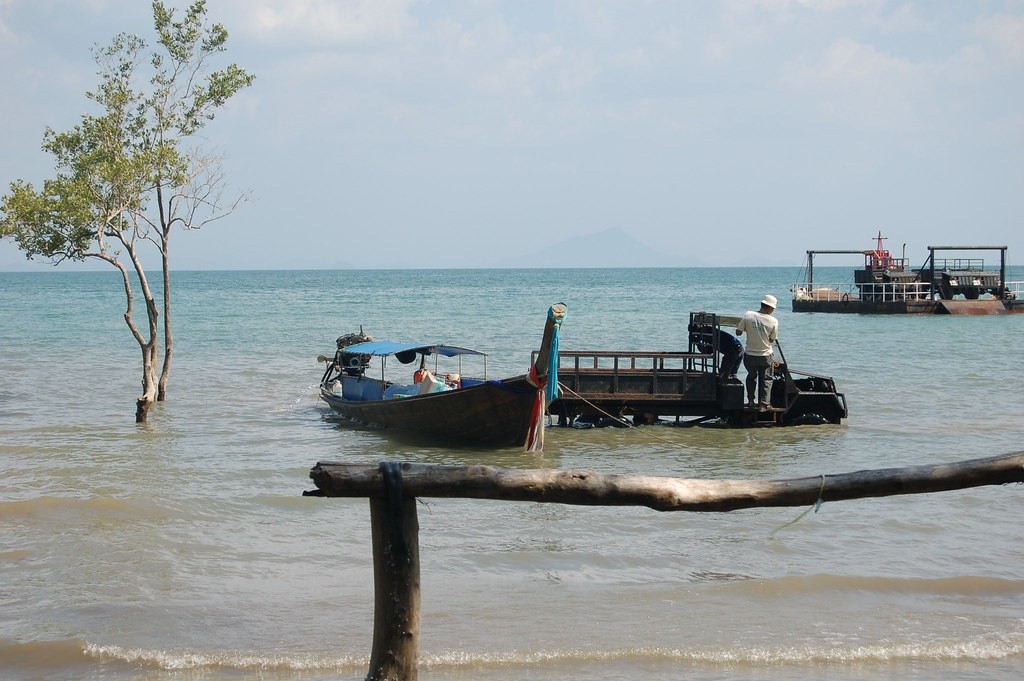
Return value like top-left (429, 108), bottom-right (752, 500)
top-left (527, 310), bottom-right (849, 428)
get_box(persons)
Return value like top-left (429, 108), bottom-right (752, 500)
top-left (736, 294), bottom-right (779, 413)
top-left (688, 323), bottom-right (744, 379)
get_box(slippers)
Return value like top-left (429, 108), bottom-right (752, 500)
top-left (760, 405), bottom-right (772, 413)
top-left (748, 403), bottom-right (754, 408)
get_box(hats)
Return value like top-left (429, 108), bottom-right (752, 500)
top-left (761, 295), bottom-right (777, 309)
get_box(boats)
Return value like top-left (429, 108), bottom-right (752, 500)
top-left (318, 325), bottom-right (547, 447)
top-left (789, 231), bottom-right (1023, 315)
